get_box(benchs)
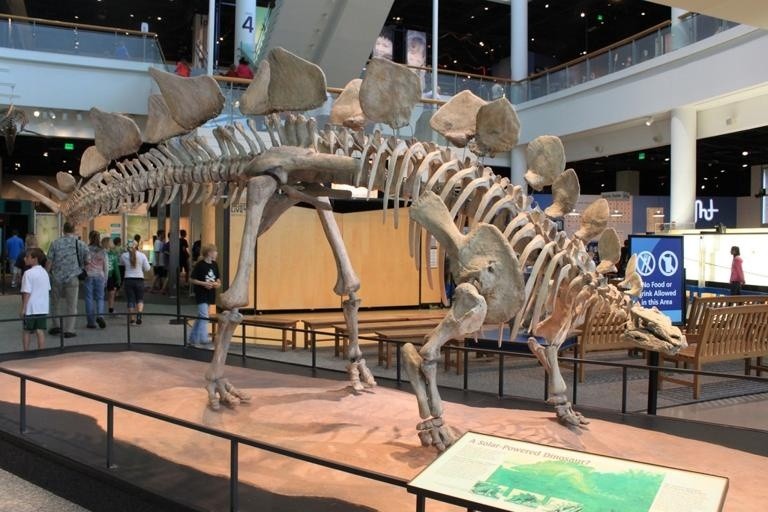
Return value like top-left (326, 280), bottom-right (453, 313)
top-left (301, 312), bottom-right (463, 376)
top-left (207, 312), bottom-right (299, 351)
top-left (561, 295), bottom-right (768, 400)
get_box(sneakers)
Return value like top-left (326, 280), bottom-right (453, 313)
top-left (129, 320), bottom-right (137, 325)
top-left (201, 344), bottom-right (215, 351)
top-left (136, 311), bottom-right (143, 324)
top-left (186, 341), bottom-right (203, 349)
top-left (96, 316), bottom-right (106, 328)
top-left (87, 323), bottom-right (97, 328)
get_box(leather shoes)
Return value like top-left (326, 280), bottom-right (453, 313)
top-left (63, 332), bottom-right (77, 338)
top-left (49, 327), bottom-right (61, 336)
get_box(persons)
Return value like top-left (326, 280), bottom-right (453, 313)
top-left (491, 80), bottom-right (504, 102)
top-left (728, 246), bottom-right (745, 306)
top-left (234, 55), bottom-right (251, 86)
top-left (19, 247), bottom-right (51, 350)
top-left (372, 27), bottom-right (392, 58)
top-left (188, 243), bottom-right (221, 347)
top-left (219, 63), bottom-right (238, 86)
top-left (0, 220), bottom-right (201, 338)
top-left (421, 65), bottom-right (444, 100)
top-left (409, 33), bottom-right (423, 87)
top-left (173, 58), bottom-right (188, 77)
top-left (585, 240), bottom-right (629, 278)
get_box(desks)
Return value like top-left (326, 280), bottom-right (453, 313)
top-left (464, 326), bottom-right (576, 403)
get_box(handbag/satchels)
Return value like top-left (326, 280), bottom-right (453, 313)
top-left (75, 239), bottom-right (87, 280)
